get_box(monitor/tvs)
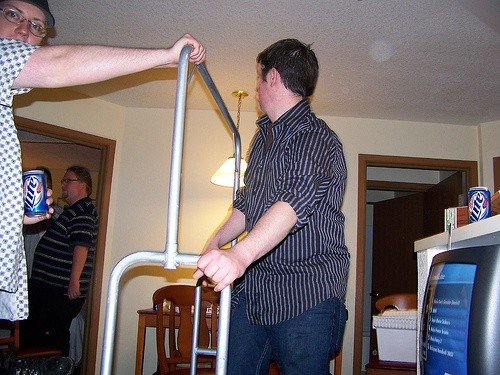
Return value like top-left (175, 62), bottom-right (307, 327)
top-left (420, 244), bottom-right (500, 375)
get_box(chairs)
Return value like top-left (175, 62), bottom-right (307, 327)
top-left (152, 284), bottom-right (220, 375)
top-left (1, 320), bottom-right (62, 359)
top-left (365, 295), bottom-right (417, 375)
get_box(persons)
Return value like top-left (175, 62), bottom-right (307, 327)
top-left (0, 0), bottom-right (207, 357)
top-left (193, 39), bottom-right (350, 375)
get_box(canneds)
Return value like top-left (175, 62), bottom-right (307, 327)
top-left (467, 186), bottom-right (491, 224)
top-left (22, 170), bottom-right (48, 218)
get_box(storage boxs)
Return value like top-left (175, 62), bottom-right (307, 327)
top-left (374, 310), bottom-right (418, 362)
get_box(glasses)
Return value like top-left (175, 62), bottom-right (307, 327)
top-left (0, 8), bottom-right (47, 39)
top-left (61, 179), bottom-right (80, 184)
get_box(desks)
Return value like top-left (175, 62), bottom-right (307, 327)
top-left (414, 213), bottom-right (499, 375)
top-left (135, 307), bottom-right (342, 375)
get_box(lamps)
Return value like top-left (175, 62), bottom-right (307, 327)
top-left (210, 155), bottom-right (248, 189)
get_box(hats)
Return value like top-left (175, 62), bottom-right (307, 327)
top-left (30, 0), bottom-right (55, 28)
